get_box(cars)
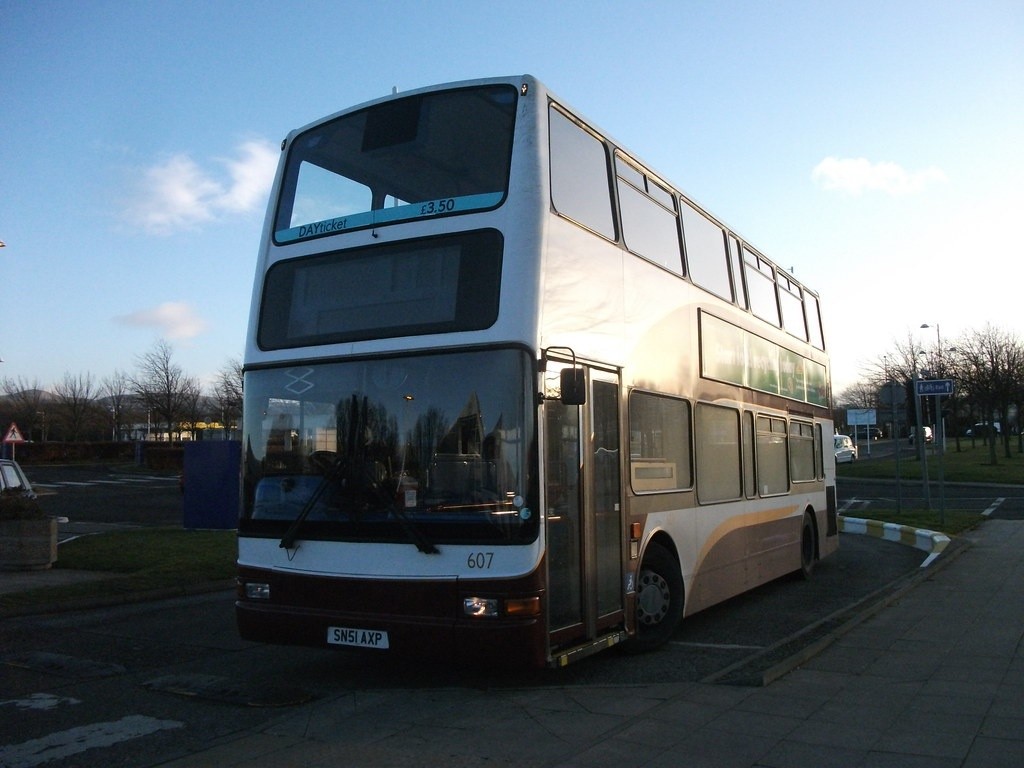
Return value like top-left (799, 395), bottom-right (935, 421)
top-left (0, 459), bottom-right (37, 502)
top-left (834, 435), bottom-right (856, 464)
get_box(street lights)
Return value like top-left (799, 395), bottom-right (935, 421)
top-left (884, 356), bottom-right (887, 384)
top-left (920, 323), bottom-right (940, 380)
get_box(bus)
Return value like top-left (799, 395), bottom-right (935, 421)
top-left (235, 73), bottom-right (838, 669)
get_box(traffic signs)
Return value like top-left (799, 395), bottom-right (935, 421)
top-left (916, 380), bottom-right (953, 395)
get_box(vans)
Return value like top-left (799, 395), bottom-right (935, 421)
top-left (966, 422), bottom-right (1000, 437)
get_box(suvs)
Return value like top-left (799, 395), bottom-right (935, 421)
top-left (909, 427), bottom-right (932, 445)
top-left (849, 428), bottom-right (883, 440)
